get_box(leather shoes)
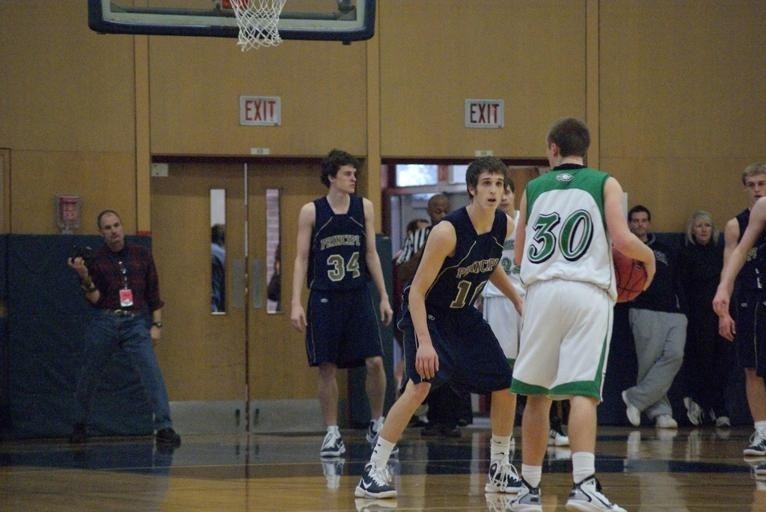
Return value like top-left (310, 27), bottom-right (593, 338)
top-left (421, 424), bottom-right (440, 438)
top-left (440, 425), bottom-right (462, 438)
top-left (70, 438), bottom-right (93, 462)
top-left (156, 427), bottom-right (182, 449)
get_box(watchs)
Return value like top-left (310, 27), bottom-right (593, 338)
top-left (151, 322), bottom-right (163, 327)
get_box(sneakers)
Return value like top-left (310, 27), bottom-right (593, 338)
top-left (504, 488), bottom-right (543, 512)
top-left (320, 436), bottom-right (346, 456)
top-left (716, 417), bottom-right (732, 428)
top-left (622, 391), bottom-right (642, 428)
top-left (744, 456), bottom-right (766, 480)
top-left (684, 397), bottom-right (704, 426)
top-left (366, 422), bottom-right (402, 454)
top-left (547, 445), bottom-right (572, 463)
top-left (656, 415), bottom-right (679, 429)
top-left (627, 432), bottom-right (642, 459)
top-left (484, 459), bottom-right (543, 497)
top-left (320, 458), bottom-right (346, 479)
top-left (548, 428), bottom-right (570, 446)
top-left (354, 498), bottom-right (399, 512)
top-left (484, 493), bottom-right (516, 511)
top-left (689, 430), bottom-right (701, 455)
top-left (716, 428), bottom-right (732, 440)
top-left (710, 408), bottom-right (716, 422)
top-left (743, 431), bottom-right (766, 456)
top-left (566, 485), bottom-right (629, 512)
top-left (356, 463), bottom-right (399, 499)
top-left (658, 431), bottom-right (674, 439)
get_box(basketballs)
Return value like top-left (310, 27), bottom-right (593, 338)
top-left (611, 246), bottom-right (647, 303)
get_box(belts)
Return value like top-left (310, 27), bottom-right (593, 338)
top-left (101, 306), bottom-right (146, 317)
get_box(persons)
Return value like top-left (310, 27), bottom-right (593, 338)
top-left (480, 179), bottom-right (570, 447)
top-left (718, 163), bottom-right (766, 456)
top-left (354, 156), bottom-right (542, 497)
top-left (290, 147), bottom-right (399, 457)
top-left (212, 224), bottom-right (248, 312)
top-left (506, 116), bottom-right (655, 512)
top-left (677, 208), bottom-right (731, 429)
top-left (620, 206), bottom-right (688, 428)
top-left (395, 194), bottom-right (461, 436)
top-left (712, 194), bottom-right (766, 475)
top-left (66, 210), bottom-right (181, 445)
top-left (391, 219), bottom-right (429, 428)
